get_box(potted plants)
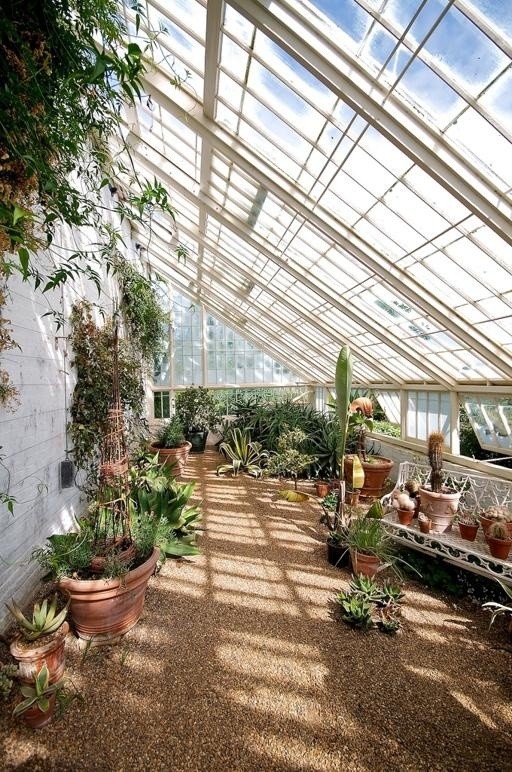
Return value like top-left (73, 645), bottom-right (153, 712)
top-left (344, 483), bottom-right (361, 508)
top-left (458, 509), bottom-right (480, 541)
top-left (343, 396), bottom-right (395, 499)
top-left (331, 510), bottom-right (423, 585)
top-left (392, 493), bottom-right (416, 526)
top-left (32, 302), bottom-right (162, 644)
top-left (3, 593), bottom-right (70, 688)
top-left (417, 430), bottom-right (460, 532)
top-left (173, 384), bottom-right (224, 456)
top-left (484, 522), bottom-right (512, 561)
top-left (147, 413), bottom-right (193, 477)
top-left (408, 477), bottom-right (420, 494)
top-left (293, 487), bottom-right (353, 568)
top-left (12, 660), bottom-right (63, 732)
top-left (419, 512), bottom-right (433, 535)
top-left (479, 506), bottom-right (512, 543)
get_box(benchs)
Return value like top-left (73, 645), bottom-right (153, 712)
top-left (379, 461), bottom-right (512, 589)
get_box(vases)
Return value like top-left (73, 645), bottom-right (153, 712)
top-left (314, 481), bottom-right (330, 498)
